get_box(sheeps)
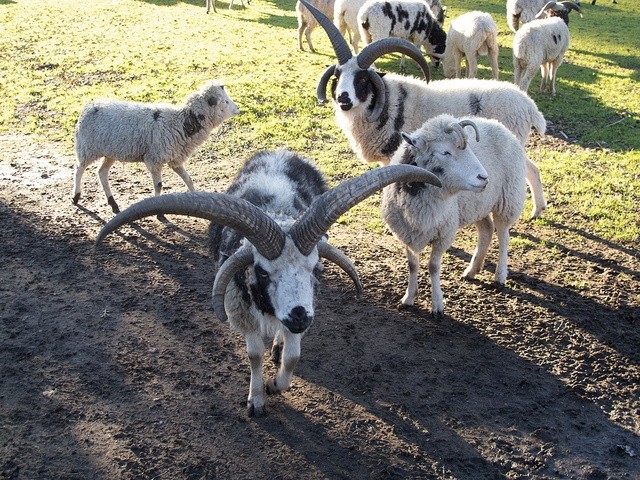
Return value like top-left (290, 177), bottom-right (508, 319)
top-left (425, 0), bottom-right (450, 27)
top-left (356, 0), bottom-right (446, 69)
top-left (74, 82), bottom-right (241, 222)
top-left (333, 0), bottom-right (367, 54)
top-left (96, 149), bottom-right (443, 420)
top-left (296, 0), bottom-right (333, 54)
top-left (419, 10), bottom-right (500, 81)
top-left (380, 113), bottom-right (527, 319)
top-left (504, 0), bottom-right (555, 33)
top-left (512, 0), bottom-right (585, 98)
top-left (300, 0), bottom-right (547, 220)
